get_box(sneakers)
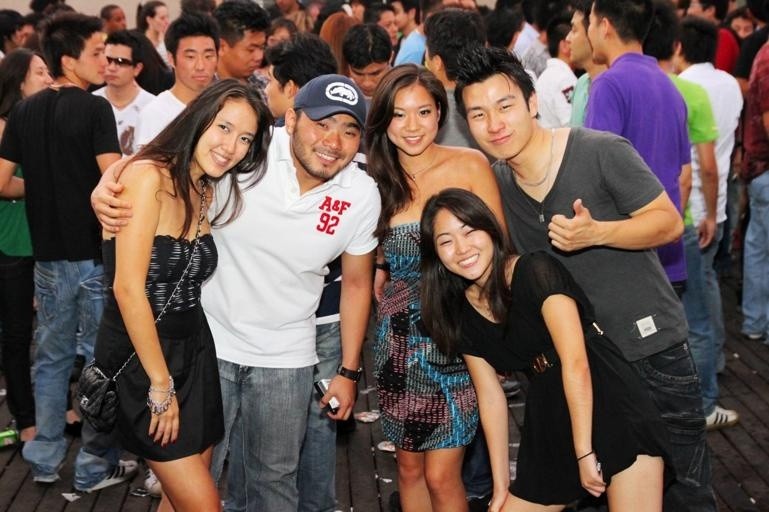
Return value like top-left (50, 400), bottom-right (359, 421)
top-left (716, 253), bottom-right (737, 266)
top-left (73, 460), bottom-right (139, 491)
top-left (706, 406), bottom-right (738, 430)
top-left (749, 332), bottom-right (763, 339)
top-left (33, 473), bottom-right (61, 485)
top-left (716, 367), bottom-right (724, 374)
top-left (19, 432), bottom-right (26, 458)
top-left (145, 468), bottom-right (162, 497)
top-left (64, 419), bottom-right (81, 438)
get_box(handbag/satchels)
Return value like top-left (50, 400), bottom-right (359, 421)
top-left (75, 178), bottom-right (206, 436)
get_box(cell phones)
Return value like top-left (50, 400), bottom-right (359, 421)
top-left (596, 462), bottom-right (603, 482)
top-left (313, 379), bottom-right (341, 414)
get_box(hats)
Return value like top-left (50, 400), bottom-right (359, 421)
top-left (294, 74), bottom-right (367, 127)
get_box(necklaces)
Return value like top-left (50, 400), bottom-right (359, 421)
top-left (516, 128), bottom-right (555, 186)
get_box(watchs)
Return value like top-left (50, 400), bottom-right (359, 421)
top-left (336, 365), bottom-right (364, 383)
top-left (372, 260), bottom-right (392, 273)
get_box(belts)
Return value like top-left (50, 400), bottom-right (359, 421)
top-left (524, 323), bottom-right (605, 381)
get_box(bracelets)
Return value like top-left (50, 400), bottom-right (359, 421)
top-left (147, 377), bottom-right (178, 413)
top-left (576, 452), bottom-right (594, 462)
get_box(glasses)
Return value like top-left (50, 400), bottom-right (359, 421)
top-left (106, 56), bottom-right (135, 67)
top-left (689, 3), bottom-right (702, 7)
top-left (267, 38), bottom-right (284, 43)
top-left (378, 21), bottom-right (398, 28)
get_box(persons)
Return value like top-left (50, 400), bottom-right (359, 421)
top-left (667, 16), bottom-right (746, 376)
top-left (373, 5), bottom-right (499, 503)
top-left (638, 2), bottom-right (743, 431)
top-left (0, 0), bottom-right (769, 155)
top-left (454, 50), bottom-right (718, 512)
top-left (96, 79), bottom-right (271, 512)
top-left (743, 40), bottom-right (769, 346)
top-left (207, 72), bottom-right (382, 512)
top-left (417, 188), bottom-right (665, 512)
top-left (368, 63), bottom-right (511, 512)
top-left (2, 15), bottom-right (137, 492)
top-left (583, 0), bottom-right (691, 307)
top-left (0, 42), bottom-right (56, 444)
